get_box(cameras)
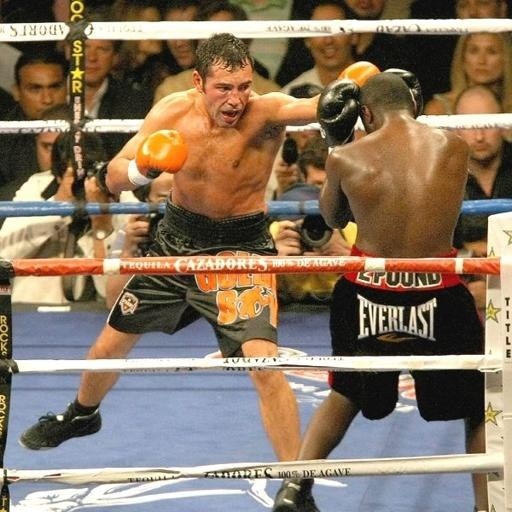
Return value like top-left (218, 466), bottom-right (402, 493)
top-left (72, 160), bottom-right (114, 201)
top-left (137, 213), bottom-right (164, 252)
top-left (297, 214), bottom-right (333, 252)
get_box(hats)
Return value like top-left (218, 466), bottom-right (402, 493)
top-left (276, 182), bottom-right (320, 221)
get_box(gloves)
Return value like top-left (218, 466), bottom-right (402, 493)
top-left (128, 129), bottom-right (187, 186)
top-left (316, 61), bottom-right (424, 155)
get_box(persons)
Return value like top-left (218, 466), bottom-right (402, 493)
top-left (1, 1), bottom-right (511, 329)
top-left (18, 32), bottom-right (381, 511)
top-left (273, 68), bottom-right (489, 511)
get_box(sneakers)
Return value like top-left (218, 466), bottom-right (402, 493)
top-left (271, 478), bottom-right (321, 512)
top-left (18, 402), bottom-right (102, 451)
